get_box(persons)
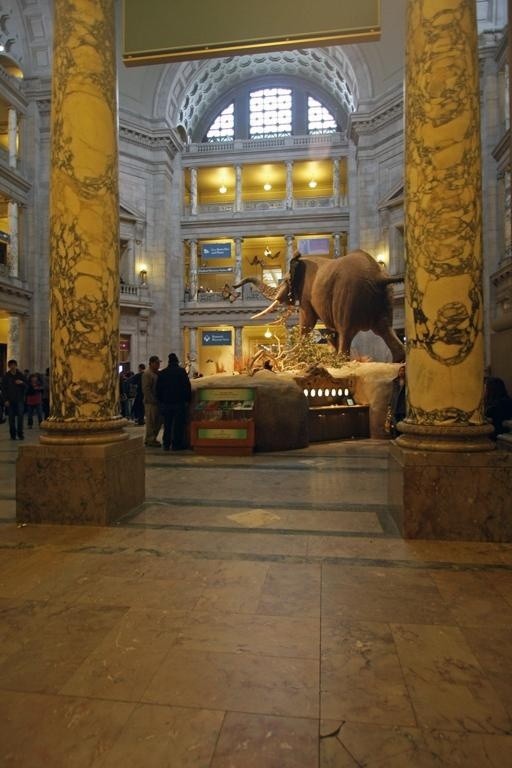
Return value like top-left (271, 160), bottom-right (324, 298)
top-left (198, 286), bottom-right (206, 293)
top-left (0, 353), bottom-right (203, 451)
top-left (387, 367), bottom-right (405, 438)
top-left (206, 288), bottom-right (213, 293)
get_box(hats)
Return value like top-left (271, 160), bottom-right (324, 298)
top-left (150, 356), bottom-right (162, 363)
top-left (168, 352), bottom-right (179, 365)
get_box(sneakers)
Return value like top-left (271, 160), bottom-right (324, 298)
top-left (147, 441), bottom-right (161, 447)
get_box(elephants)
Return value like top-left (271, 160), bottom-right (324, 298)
top-left (231, 249), bottom-right (404, 364)
